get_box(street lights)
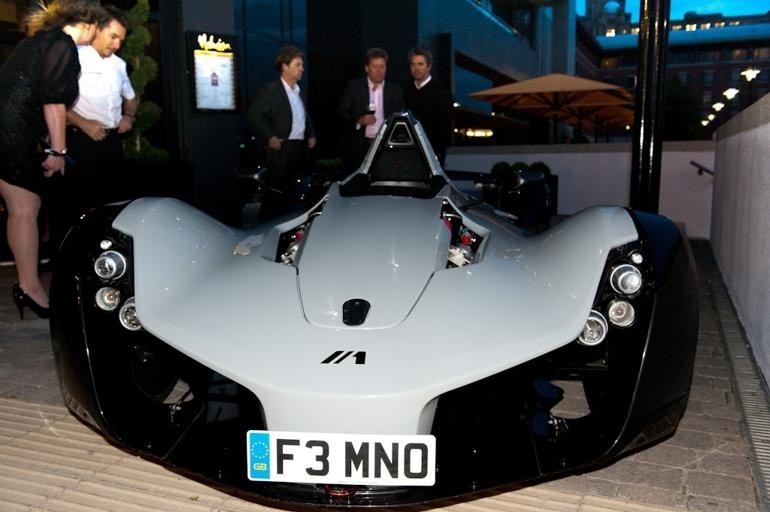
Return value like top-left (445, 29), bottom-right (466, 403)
top-left (700, 66), bottom-right (761, 137)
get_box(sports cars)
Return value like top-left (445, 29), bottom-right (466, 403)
top-left (39, 107), bottom-right (705, 510)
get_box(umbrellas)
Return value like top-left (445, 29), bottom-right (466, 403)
top-left (467, 73), bottom-right (634, 144)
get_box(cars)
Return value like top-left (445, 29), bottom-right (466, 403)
top-left (235, 165), bottom-right (270, 224)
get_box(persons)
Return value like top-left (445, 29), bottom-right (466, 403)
top-left (1, 0), bottom-right (105, 320)
top-left (402, 48), bottom-right (452, 171)
top-left (337, 48), bottom-right (401, 171)
top-left (248, 47), bottom-right (319, 218)
top-left (63, 9), bottom-right (139, 212)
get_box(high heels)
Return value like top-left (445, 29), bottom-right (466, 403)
top-left (10, 280), bottom-right (51, 322)
